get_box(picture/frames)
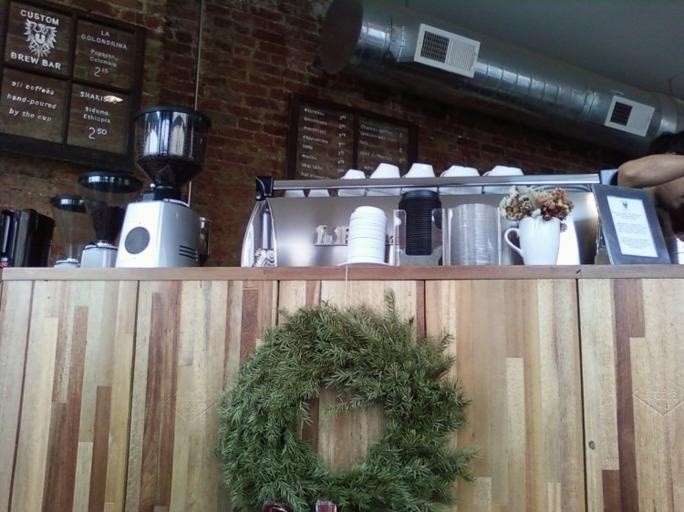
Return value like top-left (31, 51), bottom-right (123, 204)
top-left (592, 183), bottom-right (672, 264)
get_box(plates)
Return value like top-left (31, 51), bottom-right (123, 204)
top-left (336, 262), bottom-right (400, 269)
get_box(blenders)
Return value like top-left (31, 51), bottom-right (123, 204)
top-left (50, 105), bottom-right (213, 268)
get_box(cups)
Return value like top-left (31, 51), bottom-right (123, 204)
top-left (283, 162), bottom-right (528, 198)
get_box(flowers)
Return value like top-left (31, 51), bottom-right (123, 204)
top-left (499, 185), bottom-right (575, 221)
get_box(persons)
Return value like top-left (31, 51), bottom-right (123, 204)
top-left (609, 130), bottom-right (684, 242)
